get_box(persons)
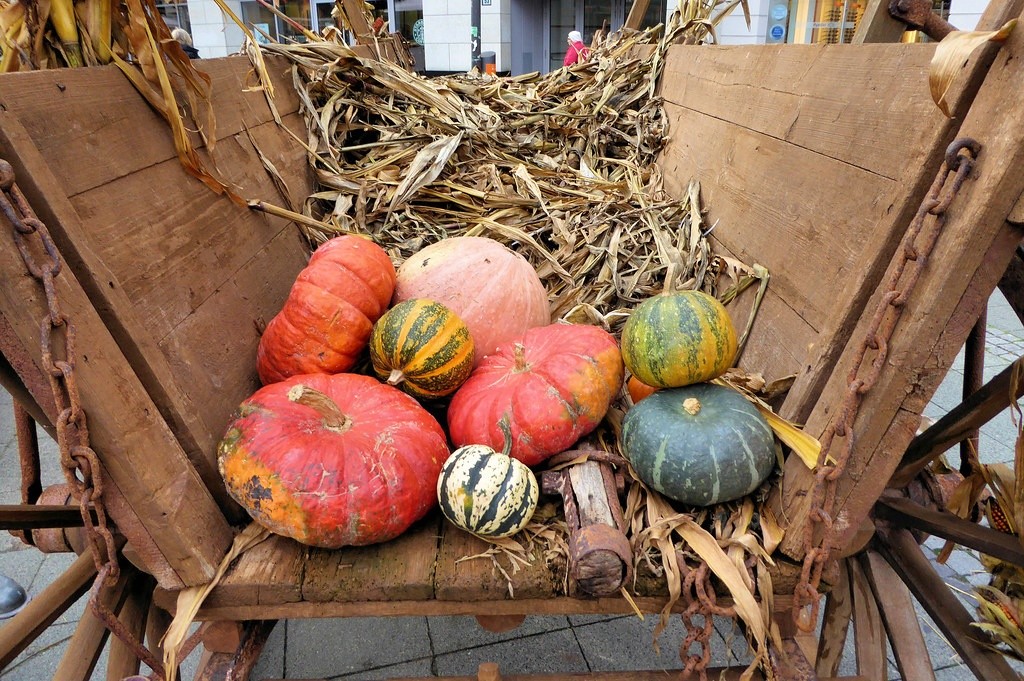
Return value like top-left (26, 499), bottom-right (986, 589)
top-left (562, 30), bottom-right (589, 69)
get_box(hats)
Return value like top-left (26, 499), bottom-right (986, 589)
top-left (567, 31), bottom-right (583, 43)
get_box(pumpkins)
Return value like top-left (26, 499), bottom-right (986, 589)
top-left (218, 231), bottom-right (775, 549)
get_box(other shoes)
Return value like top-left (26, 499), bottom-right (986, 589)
top-left (0, 574), bottom-right (31, 618)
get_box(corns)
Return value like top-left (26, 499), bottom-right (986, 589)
top-left (976, 583), bottom-right (1022, 636)
top-left (983, 494), bottom-right (1014, 544)
